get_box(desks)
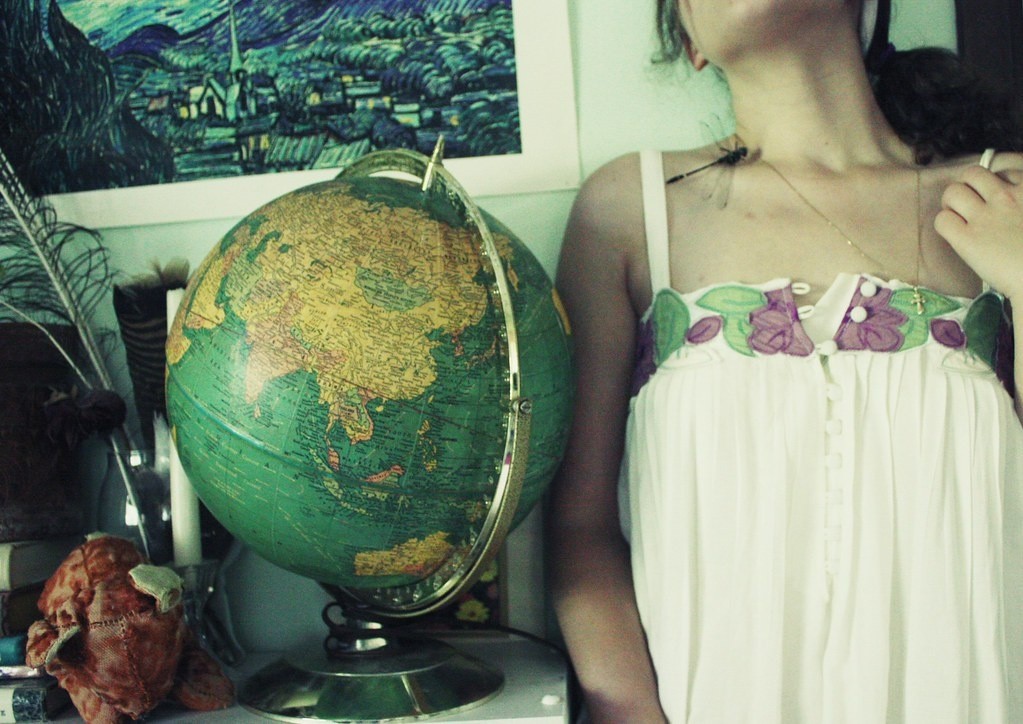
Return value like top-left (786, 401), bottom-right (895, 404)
top-left (0, 628), bottom-right (571, 724)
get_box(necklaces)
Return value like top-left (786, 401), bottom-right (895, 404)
top-left (732, 132), bottom-right (927, 316)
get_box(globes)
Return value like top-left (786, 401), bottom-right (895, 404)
top-left (163, 131), bottom-right (582, 724)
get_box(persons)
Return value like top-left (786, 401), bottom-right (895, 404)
top-left (537, 2), bottom-right (1023, 724)
